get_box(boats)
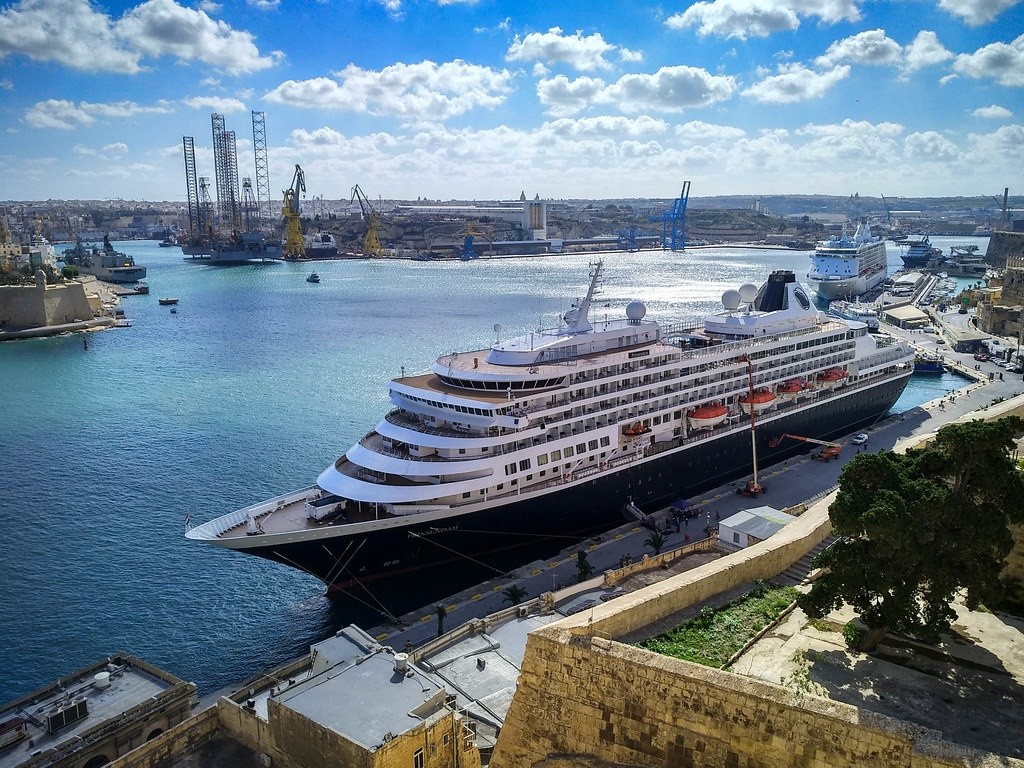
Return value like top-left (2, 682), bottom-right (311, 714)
top-left (914, 352), bottom-right (948, 375)
top-left (828, 290), bottom-right (880, 331)
top-left (305, 270), bottom-right (322, 285)
top-left (158, 297), bottom-right (179, 305)
top-left (170, 307), bottom-right (178, 314)
top-left (64, 233), bottom-right (147, 282)
top-left (900, 241), bottom-right (942, 266)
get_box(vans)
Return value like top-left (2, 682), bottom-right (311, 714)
top-left (924, 327), bottom-right (934, 333)
top-left (937, 339), bottom-right (945, 344)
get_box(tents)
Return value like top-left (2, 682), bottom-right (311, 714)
top-left (670, 500), bottom-right (694, 513)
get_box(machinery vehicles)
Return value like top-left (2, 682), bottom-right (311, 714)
top-left (768, 433), bottom-right (842, 464)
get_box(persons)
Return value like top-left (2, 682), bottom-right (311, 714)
top-left (706, 512), bottom-right (711, 525)
top-left (864, 442), bottom-right (868, 450)
top-left (857, 448), bottom-right (861, 454)
top-left (715, 510), bottom-right (720, 522)
top-left (676, 516), bottom-right (680, 533)
top-left (685, 518), bottom-right (689, 527)
top-left (975, 364), bottom-right (981, 371)
top-left (667, 505), bottom-right (703, 519)
top-left (941, 400), bottom-right (945, 412)
top-left (630, 500), bottom-right (639, 513)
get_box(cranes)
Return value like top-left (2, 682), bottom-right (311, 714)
top-left (348, 184), bottom-right (385, 259)
top-left (183, 108), bottom-right (283, 252)
top-left (281, 163), bottom-right (308, 260)
top-left (460, 222), bottom-right (496, 263)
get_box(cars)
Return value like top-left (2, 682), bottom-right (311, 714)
top-left (974, 353), bottom-right (1020, 372)
top-left (959, 309), bottom-right (967, 314)
top-left (852, 433), bottom-right (869, 444)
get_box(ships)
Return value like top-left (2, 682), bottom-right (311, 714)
top-left (181, 258), bottom-right (918, 621)
top-left (805, 221), bottom-right (887, 300)
